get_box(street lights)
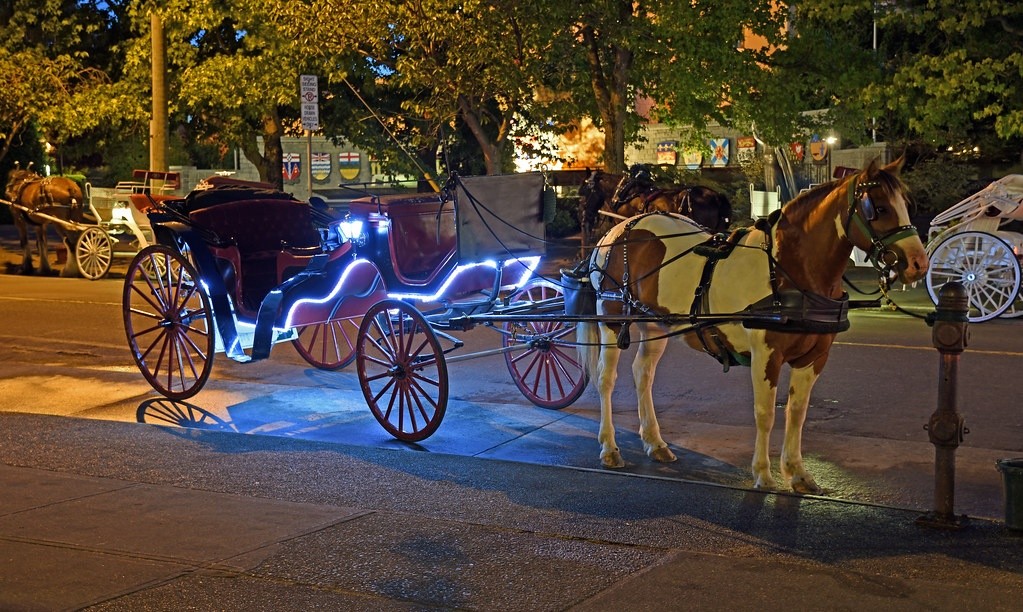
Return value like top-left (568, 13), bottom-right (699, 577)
top-left (821, 130), bottom-right (838, 183)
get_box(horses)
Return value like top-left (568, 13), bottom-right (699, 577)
top-left (5, 169), bottom-right (83, 278)
top-left (575, 152), bottom-right (928, 495)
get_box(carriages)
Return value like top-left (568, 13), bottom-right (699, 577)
top-left (121, 147), bottom-right (933, 496)
top-left (579, 161), bottom-right (1023, 323)
top-left (0, 165), bottom-right (199, 284)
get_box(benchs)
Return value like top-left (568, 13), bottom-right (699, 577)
top-left (189, 197), bottom-right (316, 318)
top-left (128, 193), bottom-right (186, 223)
top-left (340, 177), bottom-right (455, 205)
top-left (276, 201), bottom-right (386, 287)
top-left (964, 199), bottom-right (1002, 234)
